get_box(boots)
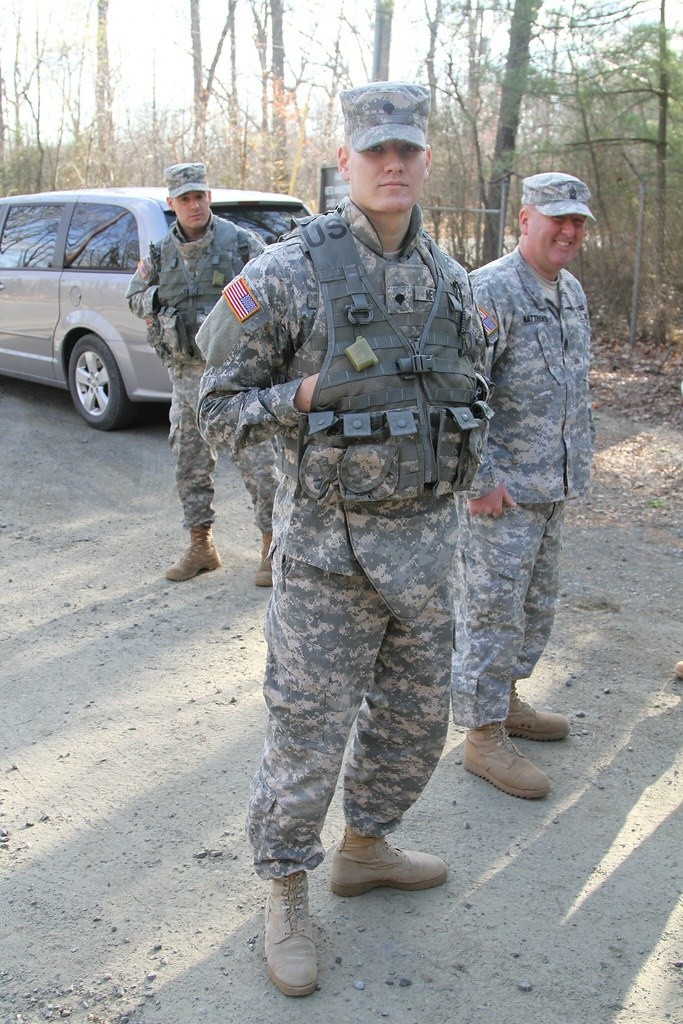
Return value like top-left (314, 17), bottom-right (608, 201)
top-left (265, 870), bottom-right (318, 995)
top-left (463, 722), bottom-right (550, 799)
top-left (255, 532), bottom-right (273, 586)
top-left (504, 679), bottom-right (570, 742)
top-left (330, 824), bottom-right (447, 897)
top-left (166, 524), bottom-right (221, 581)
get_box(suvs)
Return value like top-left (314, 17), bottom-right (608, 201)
top-left (0, 187), bottom-right (311, 433)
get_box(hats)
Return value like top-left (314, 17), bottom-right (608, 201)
top-left (164, 162), bottom-right (211, 197)
top-left (340, 81), bottom-right (431, 153)
top-left (521, 171), bottom-right (596, 222)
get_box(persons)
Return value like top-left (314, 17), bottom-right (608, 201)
top-left (125, 162), bottom-right (283, 585)
top-left (197, 81), bottom-right (495, 999)
top-left (456, 172), bottom-right (597, 798)
